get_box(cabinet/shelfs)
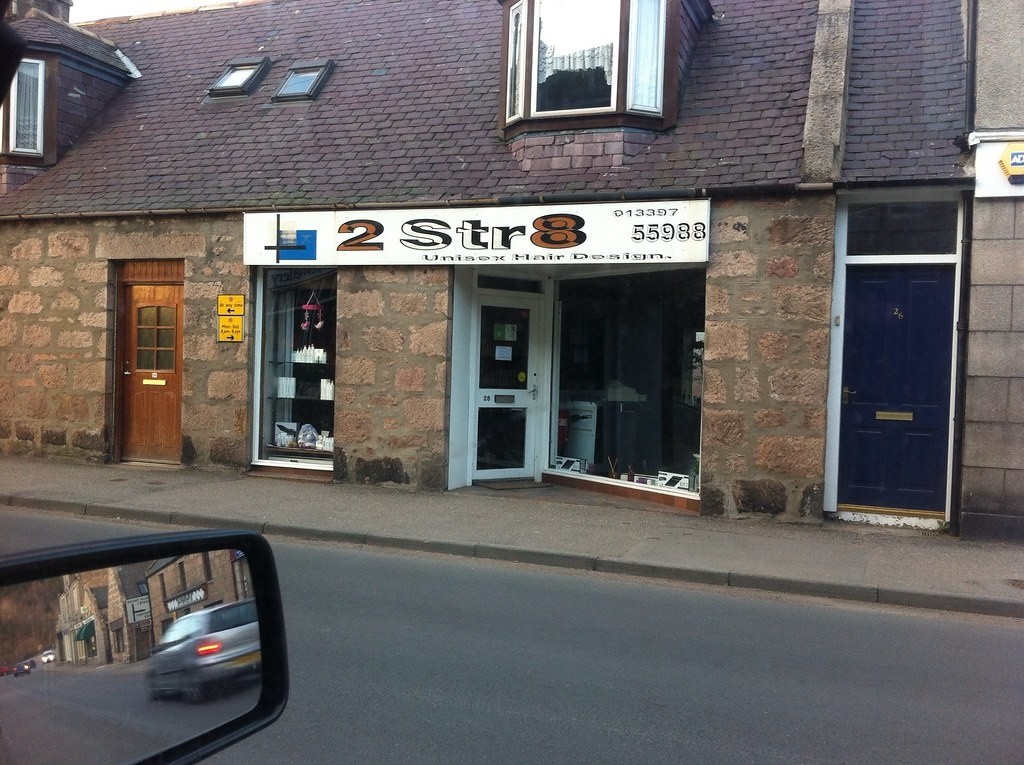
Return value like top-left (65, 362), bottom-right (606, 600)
top-left (265, 361), bottom-right (335, 460)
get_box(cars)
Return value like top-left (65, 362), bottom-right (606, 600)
top-left (136, 597), bottom-right (262, 706)
top-left (13, 663), bottom-right (31, 677)
top-left (24, 660), bottom-right (37, 670)
top-left (0, 666), bottom-right (9, 676)
top-left (42, 650), bottom-right (56, 664)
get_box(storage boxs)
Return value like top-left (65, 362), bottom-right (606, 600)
top-left (554, 456), bottom-right (586, 474)
top-left (657, 471), bottom-right (690, 491)
